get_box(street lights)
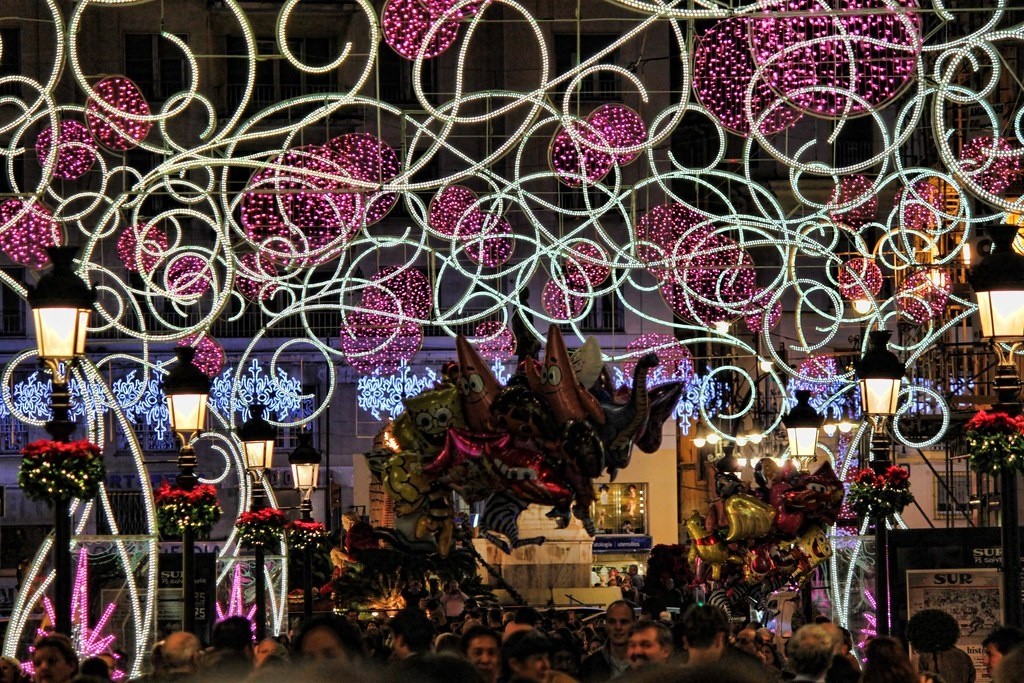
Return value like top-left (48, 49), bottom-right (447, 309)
top-left (781, 390), bottom-right (824, 623)
top-left (160, 345), bottom-right (214, 635)
top-left (236, 403), bottom-right (278, 644)
top-left (854, 329), bottom-right (905, 636)
top-left (964, 223), bottom-right (1024, 627)
top-left (288, 431), bottom-right (322, 619)
top-left (26, 244), bottom-right (98, 636)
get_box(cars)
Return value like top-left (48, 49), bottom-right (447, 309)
top-left (766, 536), bottom-right (876, 628)
top-left (539, 606), bottom-right (684, 638)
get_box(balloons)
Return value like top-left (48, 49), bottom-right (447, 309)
top-left (363, 322), bottom-right (685, 561)
top-left (687, 459), bottom-right (843, 590)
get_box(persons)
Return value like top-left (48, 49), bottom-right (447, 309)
top-left (0, 565), bottom-right (1024, 683)
